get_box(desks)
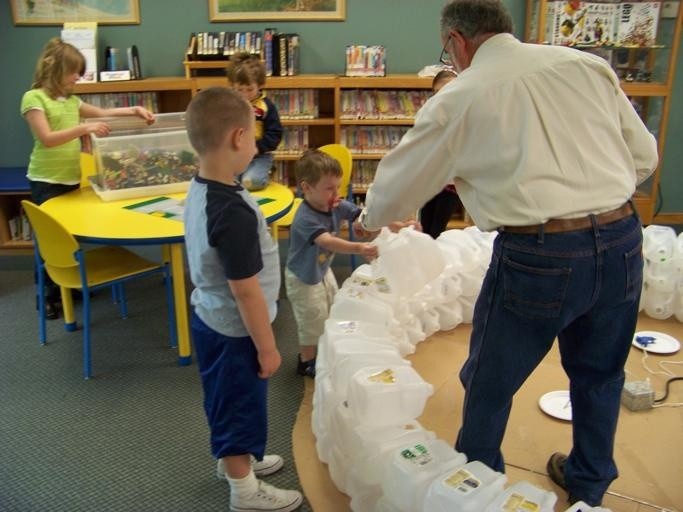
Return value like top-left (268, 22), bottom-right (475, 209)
top-left (40, 180), bottom-right (295, 366)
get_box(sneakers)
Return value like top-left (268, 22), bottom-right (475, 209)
top-left (35, 295), bottom-right (60, 320)
top-left (228, 481), bottom-right (304, 512)
top-left (216, 453), bottom-right (284, 481)
top-left (295, 352), bottom-right (316, 379)
top-left (546, 452), bottom-right (602, 509)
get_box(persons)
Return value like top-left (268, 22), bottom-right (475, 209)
top-left (181, 84), bottom-right (306, 512)
top-left (413, 69), bottom-right (459, 244)
top-left (225, 52), bottom-right (285, 193)
top-left (283, 148), bottom-right (425, 378)
top-left (17, 36), bottom-right (157, 322)
top-left (347, 0), bottom-right (661, 512)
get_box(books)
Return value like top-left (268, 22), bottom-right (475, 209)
top-left (260, 87), bottom-right (437, 233)
top-left (60, 21), bottom-right (98, 85)
top-left (525, 0), bottom-right (662, 83)
top-left (341, 42), bottom-right (387, 77)
top-left (628, 96), bottom-right (648, 122)
top-left (180, 24), bottom-right (298, 80)
top-left (72, 90), bottom-right (159, 156)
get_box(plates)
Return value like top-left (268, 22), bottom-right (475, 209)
top-left (537, 388), bottom-right (574, 422)
top-left (631, 329), bottom-right (681, 354)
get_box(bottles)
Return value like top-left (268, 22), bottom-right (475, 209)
top-left (104, 47), bottom-right (119, 71)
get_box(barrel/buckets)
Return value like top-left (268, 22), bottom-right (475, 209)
top-left (310, 221), bottom-right (683, 511)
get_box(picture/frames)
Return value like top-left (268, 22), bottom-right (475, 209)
top-left (208, 0), bottom-right (346, 23)
top-left (10, 0), bottom-right (141, 26)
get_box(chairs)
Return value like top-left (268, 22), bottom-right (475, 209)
top-left (21, 199), bottom-right (178, 380)
top-left (272, 144), bottom-right (356, 273)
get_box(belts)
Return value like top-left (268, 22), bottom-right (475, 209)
top-left (497, 197), bottom-right (638, 236)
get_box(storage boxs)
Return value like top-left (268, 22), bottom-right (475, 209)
top-left (85, 111), bottom-right (200, 202)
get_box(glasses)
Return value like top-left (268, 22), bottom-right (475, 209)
top-left (440, 35), bottom-right (454, 66)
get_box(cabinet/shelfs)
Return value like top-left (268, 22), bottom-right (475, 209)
top-left (0, 167), bottom-right (36, 255)
top-left (67, 74), bottom-right (472, 239)
top-left (523, 0), bottom-right (683, 226)
